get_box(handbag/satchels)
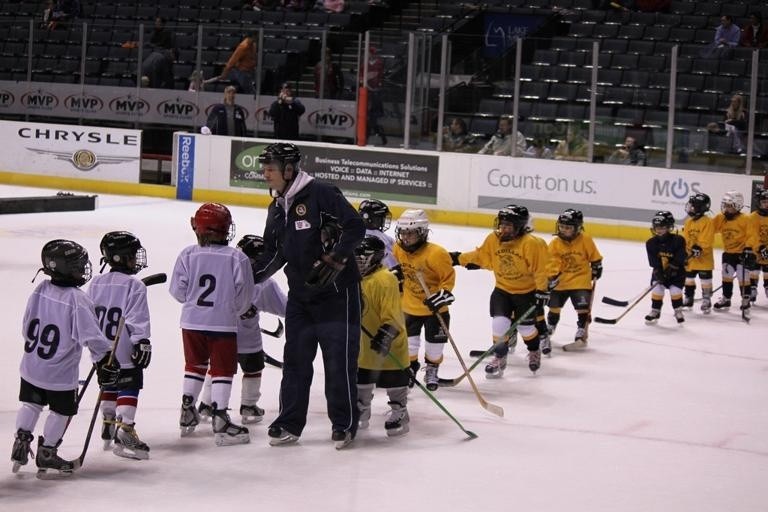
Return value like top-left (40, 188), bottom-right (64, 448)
top-left (122, 41), bottom-right (139, 48)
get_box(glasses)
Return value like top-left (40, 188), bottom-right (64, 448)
top-left (155, 22), bottom-right (161, 24)
top-left (281, 89), bottom-right (289, 92)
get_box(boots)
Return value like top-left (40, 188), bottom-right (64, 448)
top-left (359, 356), bottom-right (444, 429)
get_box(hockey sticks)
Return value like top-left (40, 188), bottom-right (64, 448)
top-left (594, 275), bottom-right (661, 324)
top-left (601, 282), bottom-right (656, 307)
top-left (60, 273), bottom-right (167, 443)
top-left (742, 258), bottom-right (750, 322)
top-left (693, 275), bottom-right (741, 301)
top-left (436, 305), bottom-right (538, 386)
top-left (259, 318), bottom-right (284, 336)
top-left (68, 318), bottom-right (125, 468)
top-left (262, 351), bottom-right (283, 368)
top-left (361, 324), bottom-right (477, 440)
top-left (471, 350), bottom-right (488, 357)
top-left (416, 271), bottom-right (504, 417)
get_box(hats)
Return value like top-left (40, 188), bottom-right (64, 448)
top-left (225, 86), bottom-right (235, 92)
top-left (281, 83), bottom-right (291, 90)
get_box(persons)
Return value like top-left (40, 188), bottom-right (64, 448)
top-left (186, 68), bottom-right (219, 92)
top-left (253, 142), bottom-right (366, 450)
top-left (353, 197), bottom-right (455, 436)
top-left (449, 204), bottom-right (602, 378)
top-left (11, 203), bottom-right (287, 478)
top-left (714, 12), bottom-right (768, 47)
top-left (314, 46), bottom-right (344, 100)
top-left (149, 16), bottom-right (175, 49)
top-left (358, 46), bottom-right (383, 88)
top-left (43, 0), bottom-right (59, 23)
top-left (221, 20), bottom-right (262, 93)
top-left (708, 92), bottom-right (750, 136)
top-left (443, 115), bottom-right (589, 161)
top-left (200, 84), bottom-right (246, 138)
top-left (131, 45), bottom-right (181, 87)
top-left (607, 135), bottom-right (648, 167)
top-left (267, 83), bottom-right (306, 140)
top-left (644, 190), bottom-right (768, 324)
top-left (248, 0), bottom-right (346, 14)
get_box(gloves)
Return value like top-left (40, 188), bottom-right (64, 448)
top-left (98, 351), bottom-right (121, 387)
top-left (308, 254), bottom-right (344, 289)
top-left (130, 338), bottom-right (153, 369)
top-left (422, 289), bottom-right (455, 314)
top-left (652, 244), bottom-right (768, 281)
top-left (448, 251), bottom-right (461, 267)
top-left (534, 289), bottom-right (551, 309)
top-left (240, 304), bottom-right (259, 321)
top-left (389, 266), bottom-right (405, 296)
top-left (548, 272), bottom-right (561, 288)
top-left (590, 259), bottom-right (602, 279)
top-left (370, 325), bottom-right (399, 355)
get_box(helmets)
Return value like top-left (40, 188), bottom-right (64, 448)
top-left (192, 203), bottom-right (231, 244)
top-left (499, 204), bottom-right (527, 236)
top-left (652, 189), bottom-right (768, 237)
top-left (394, 209), bottom-right (430, 232)
top-left (236, 235), bottom-right (263, 266)
top-left (358, 200), bottom-right (388, 231)
top-left (354, 235), bottom-right (385, 268)
top-left (256, 143), bottom-right (302, 164)
top-left (41, 240), bottom-right (87, 285)
top-left (99, 231), bottom-right (141, 274)
top-left (558, 209), bottom-right (583, 238)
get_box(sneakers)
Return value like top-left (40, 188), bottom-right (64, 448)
top-left (268, 427), bottom-right (287, 437)
top-left (11, 429), bottom-right (33, 466)
top-left (211, 402), bottom-right (250, 435)
top-left (179, 393), bottom-right (202, 426)
top-left (714, 285), bottom-right (768, 321)
top-left (35, 436), bottom-right (74, 471)
top-left (197, 402), bottom-right (214, 418)
top-left (101, 413), bottom-right (115, 439)
top-left (331, 426), bottom-right (357, 440)
top-left (114, 416), bottom-right (150, 451)
top-left (485, 325), bottom-right (588, 374)
top-left (645, 293), bottom-right (712, 322)
top-left (240, 406), bottom-right (265, 417)
